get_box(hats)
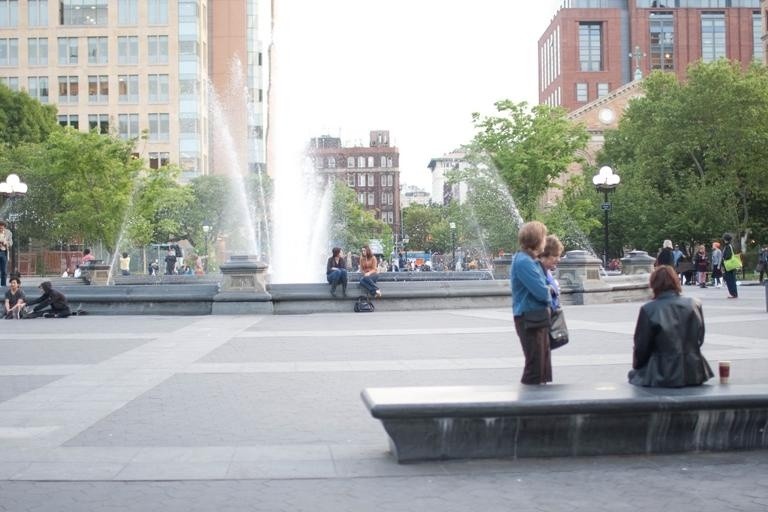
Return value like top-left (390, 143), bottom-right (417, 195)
top-left (723, 234), bottom-right (732, 241)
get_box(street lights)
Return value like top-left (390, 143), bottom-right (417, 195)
top-left (403, 233), bottom-right (410, 261)
top-left (0, 173), bottom-right (29, 282)
top-left (648, 13), bottom-right (665, 72)
top-left (199, 220), bottom-right (213, 273)
top-left (593, 165), bottom-right (620, 269)
top-left (449, 221), bottom-right (458, 268)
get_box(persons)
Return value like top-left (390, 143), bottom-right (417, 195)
top-left (654, 234), bottom-right (738, 298)
top-left (0, 221), bottom-right (13, 286)
top-left (62, 245), bottom-right (190, 278)
top-left (758, 244), bottom-right (768, 283)
top-left (629, 266), bottom-right (714, 388)
top-left (511, 221), bottom-right (556, 386)
top-left (4, 278), bottom-right (27, 319)
top-left (536, 235), bottom-right (563, 317)
top-left (327, 246), bottom-right (480, 299)
top-left (20, 281), bottom-right (72, 318)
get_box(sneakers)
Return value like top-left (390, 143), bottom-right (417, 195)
top-left (728, 294), bottom-right (737, 298)
top-left (374, 290), bottom-right (381, 298)
top-left (42, 312), bottom-right (51, 318)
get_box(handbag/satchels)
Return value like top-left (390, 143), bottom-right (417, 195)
top-left (548, 309), bottom-right (568, 349)
top-left (354, 298), bottom-right (374, 312)
top-left (756, 261), bottom-right (764, 272)
top-left (723, 253), bottom-right (743, 272)
top-left (522, 307), bottom-right (550, 329)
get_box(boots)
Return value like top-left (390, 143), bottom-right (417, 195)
top-left (343, 278), bottom-right (348, 297)
top-left (331, 281), bottom-right (338, 297)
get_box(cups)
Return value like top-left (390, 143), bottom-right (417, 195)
top-left (718, 360), bottom-right (732, 383)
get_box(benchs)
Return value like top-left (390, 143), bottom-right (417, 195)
top-left (361, 374), bottom-right (768, 464)
